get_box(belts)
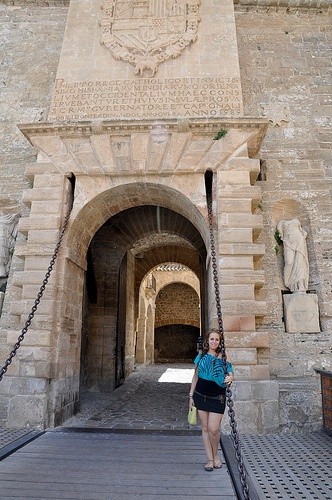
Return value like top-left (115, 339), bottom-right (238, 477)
top-left (194, 390), bottom-right (226, 403)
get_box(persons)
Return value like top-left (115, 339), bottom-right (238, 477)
top-left (182, 328), bottom-right (234, 471)
top-left (277, 218), bottom-right (309, 294)
top-left (197, 333), bottom-right (202, 356)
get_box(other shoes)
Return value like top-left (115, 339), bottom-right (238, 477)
top-left (214, 459), bottom-right (222, 469)
top-left (205, 460), bottom-right (213, 471)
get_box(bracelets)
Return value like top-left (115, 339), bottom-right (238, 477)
top-left (188, 392), bottom-right (194, 399)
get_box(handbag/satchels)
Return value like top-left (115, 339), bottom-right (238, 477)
top-left (188, 406), bottom-right (197, 425)
